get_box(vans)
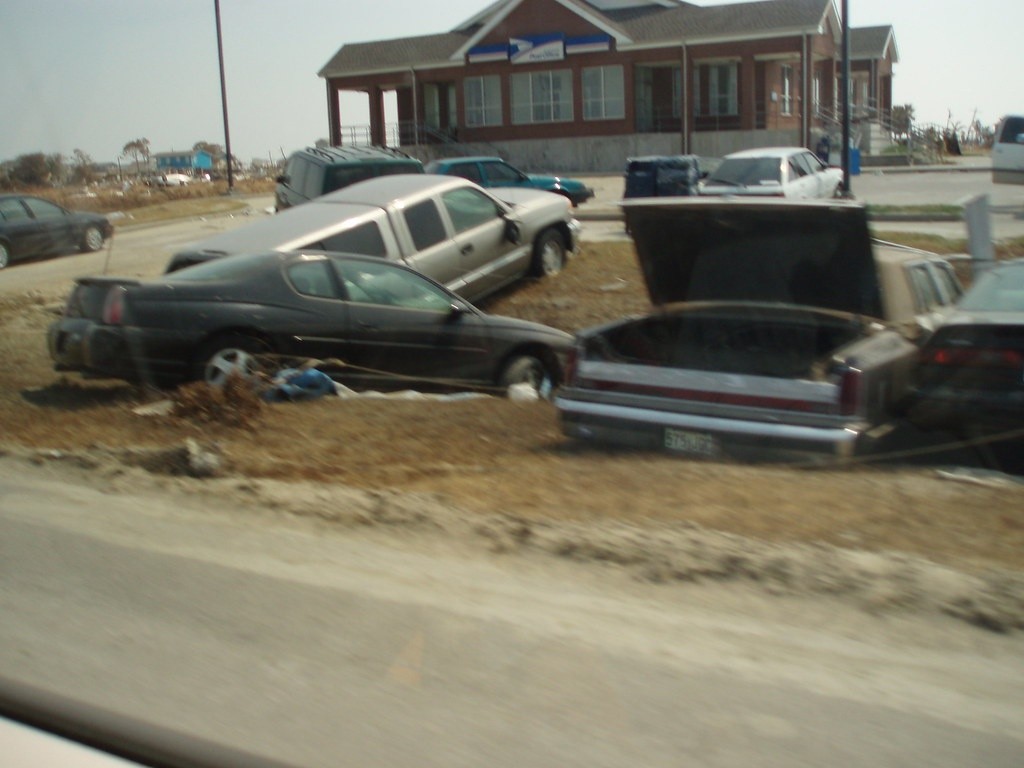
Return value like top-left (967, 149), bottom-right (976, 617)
top-left (165, 174), bottom-right (582, 303)
top-left (555, 196), bottom-right (966, 469)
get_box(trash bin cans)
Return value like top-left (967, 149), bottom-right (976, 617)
top-left (817, 134), bottom-right (860, 175)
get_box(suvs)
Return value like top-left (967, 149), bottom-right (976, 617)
top-left (624, 155), bottom-right (723, 235)
top-left (274, 144), bottom-right (426, 213)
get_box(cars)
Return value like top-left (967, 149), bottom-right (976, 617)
top-left (0, 194), bottom-right (114, 269)
top-left (698, 146), bottom-right (843, 199)
top-left (424, 156), bottom-right (595, 208)
top-left (895, 260), bottom-right (1024, 466)
top-left (47, 250), bottom-right (575, 400)
